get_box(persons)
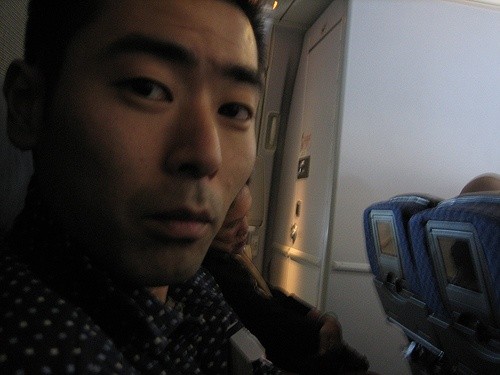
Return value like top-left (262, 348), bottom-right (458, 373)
top-left (461, 174), bottom-right (500, 203)
top-left (0, 0), bottom-right (284, 375)
top-left (202, 179), bottom-right (370, 375)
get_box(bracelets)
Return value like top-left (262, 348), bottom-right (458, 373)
top-left (315, 310), bottom-right (339, 325)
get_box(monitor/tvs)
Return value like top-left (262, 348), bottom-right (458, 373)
top-left (374, 215), bottom-right (401, 278)
top-left (431, 229), bottom-right (491, 316)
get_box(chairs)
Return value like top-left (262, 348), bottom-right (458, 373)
top-left (362, 192), bottom-right (500, 375)
